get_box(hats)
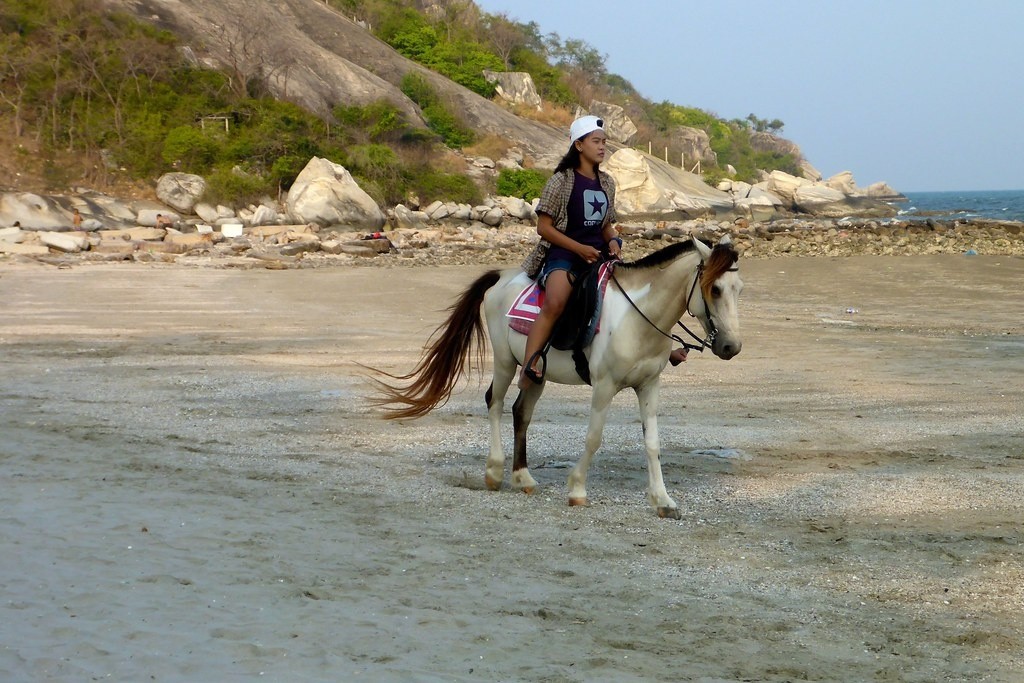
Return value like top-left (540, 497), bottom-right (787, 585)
top-left (568, 116), bottom-right (605, 151)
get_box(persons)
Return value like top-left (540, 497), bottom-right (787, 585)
top-left (518, 116), bottom-right (687, 391)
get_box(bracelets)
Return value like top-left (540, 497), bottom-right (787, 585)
top-left (609, 238), bottom-right (622, 249)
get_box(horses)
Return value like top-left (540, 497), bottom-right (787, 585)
top-left (354, 234), bottom-right (746, 522)
